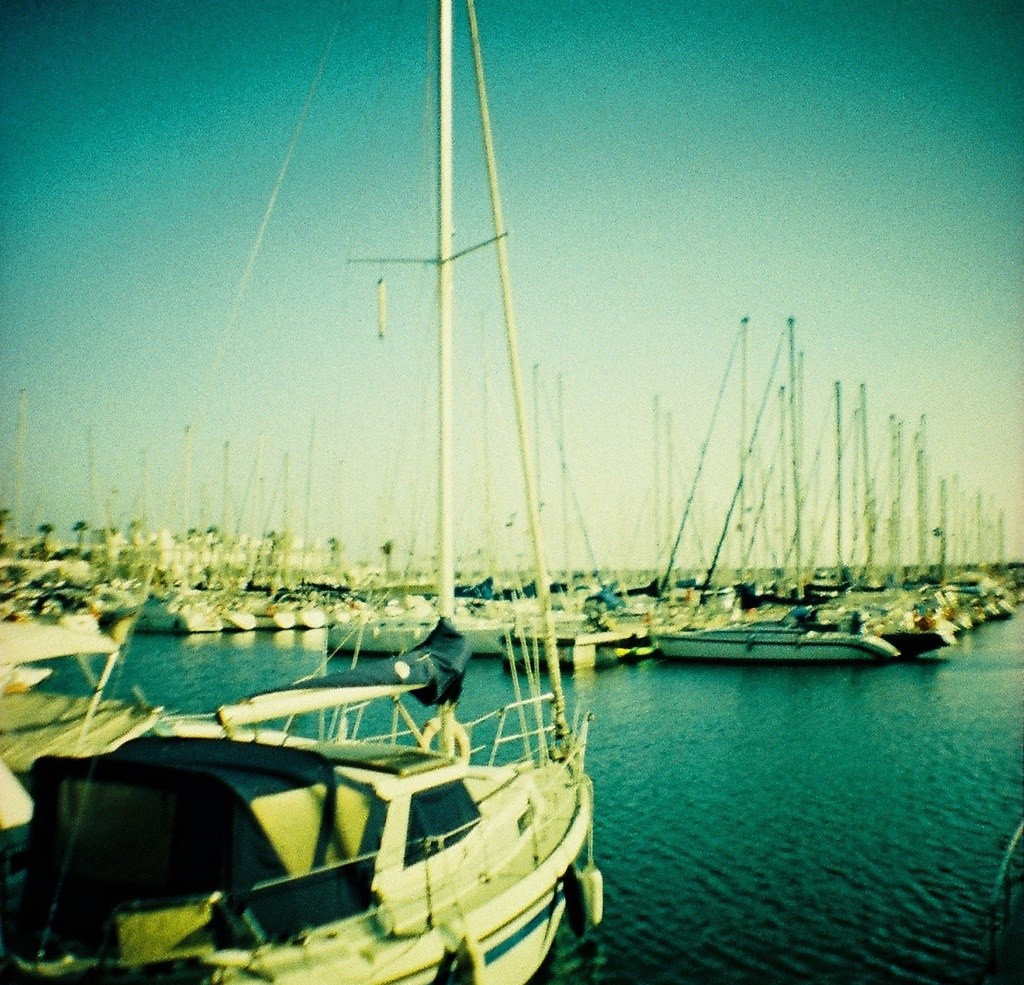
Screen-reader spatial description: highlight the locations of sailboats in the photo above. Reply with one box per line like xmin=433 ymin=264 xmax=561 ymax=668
xmin=0 ymin=2 xmax=1022 ymax=985
